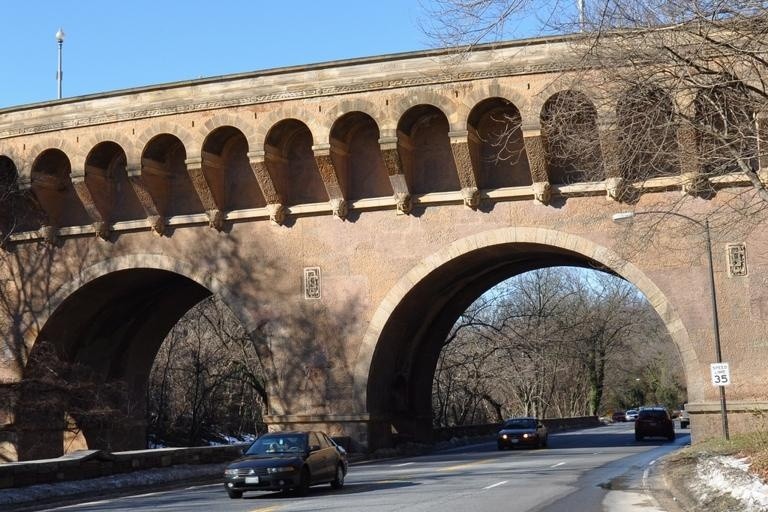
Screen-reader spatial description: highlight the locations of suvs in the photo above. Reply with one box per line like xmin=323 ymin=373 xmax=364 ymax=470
xmin=633 ymin=407 xmax=676 ymax=441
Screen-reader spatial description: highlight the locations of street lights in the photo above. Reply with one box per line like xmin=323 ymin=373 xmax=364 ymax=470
xmin=609 ymin=208 xmax=730 ymax=443
xmin=55 ymin=26 xmax=65 ymax=101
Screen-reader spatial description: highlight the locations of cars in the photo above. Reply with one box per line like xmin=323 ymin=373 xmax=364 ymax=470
xmin=496 ymin=417 xmax=549 ymax=451
xmin=612 ymin=403 xmax=691 ymax=429
xmin=224 ymin=430 xmax=349 ymax=499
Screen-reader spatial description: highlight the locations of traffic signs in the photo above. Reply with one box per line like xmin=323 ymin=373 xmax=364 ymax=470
xmin=708 ymin=362 xmax=730 ymax=387
